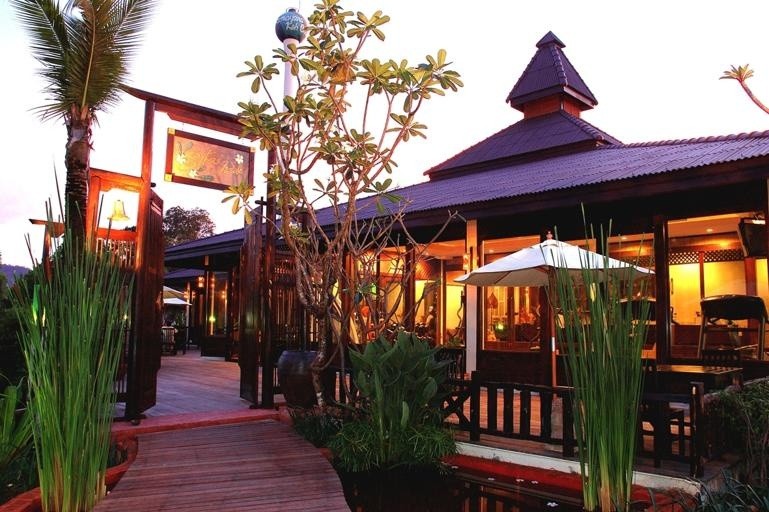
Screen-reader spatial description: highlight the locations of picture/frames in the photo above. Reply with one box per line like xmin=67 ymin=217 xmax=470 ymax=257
xmin=167 ymin=127 xmax=256 ymax=194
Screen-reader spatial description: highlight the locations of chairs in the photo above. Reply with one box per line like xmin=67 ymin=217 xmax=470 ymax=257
xmin=639 ymin=359 xmax=687 ymax=462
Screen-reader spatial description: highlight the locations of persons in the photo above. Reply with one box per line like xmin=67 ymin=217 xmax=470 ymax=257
xmin=161 ymin=321 xmax=179 ymax=354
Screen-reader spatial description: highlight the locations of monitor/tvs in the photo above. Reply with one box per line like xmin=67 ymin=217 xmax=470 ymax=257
xmin=738 ymin=216 xmax=767 ymax=258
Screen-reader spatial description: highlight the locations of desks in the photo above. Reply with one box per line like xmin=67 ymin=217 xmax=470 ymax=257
xmin=641 ymin=363 xmax=745 ymax=392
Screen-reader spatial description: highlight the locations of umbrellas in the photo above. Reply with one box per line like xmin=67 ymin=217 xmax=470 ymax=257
xmin=163 ymin=298 xmax=192 ymax=306
xmin=163 ymin=285 xmax=187 ymax=298
xmin=452 ymin=229 xmax=656 ymax=401
